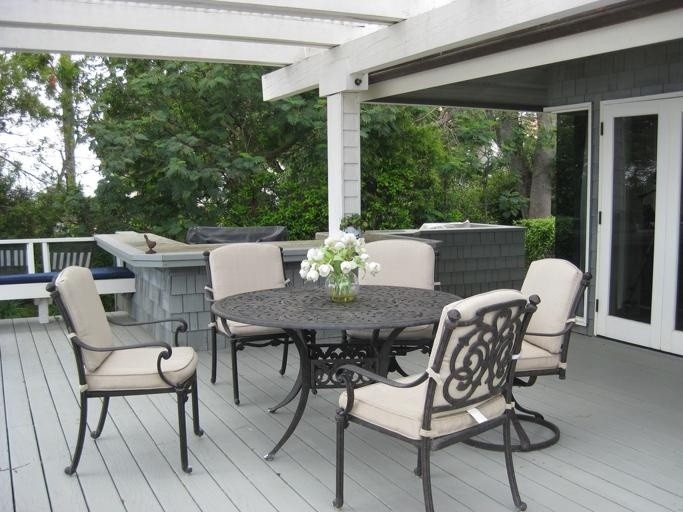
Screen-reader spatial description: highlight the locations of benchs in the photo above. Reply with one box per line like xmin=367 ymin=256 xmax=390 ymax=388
xmin=0 ymin=234 xmax=136 ymax=324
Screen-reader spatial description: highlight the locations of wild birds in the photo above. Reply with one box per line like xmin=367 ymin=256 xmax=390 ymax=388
xmin=144 ymin=235 xmax=157 ymax=253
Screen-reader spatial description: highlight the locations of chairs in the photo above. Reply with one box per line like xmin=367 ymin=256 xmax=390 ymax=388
xmin=330 ymin=287 xmax=540 ymax=512
xmin=457 ymin=256 xmax=594 ymax=453
xmin=48 ymin=264 xmax=206 ymax=477
xmin=202 ymin=242 xmax=318 ymax=405
xmin=342 ymin=235 xmax=436 ymax=378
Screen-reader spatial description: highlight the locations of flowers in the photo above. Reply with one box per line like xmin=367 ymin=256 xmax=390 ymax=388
xmin=300 ymin=232 xmax=381 ymax=303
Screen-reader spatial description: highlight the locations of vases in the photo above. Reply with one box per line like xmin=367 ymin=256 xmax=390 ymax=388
xmin=323 ymin=273 xmax=359 ymax=303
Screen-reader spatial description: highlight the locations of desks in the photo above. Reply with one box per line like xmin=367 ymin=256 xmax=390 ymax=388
xmin=203 ymin=283 xmax=465 ymax=461
